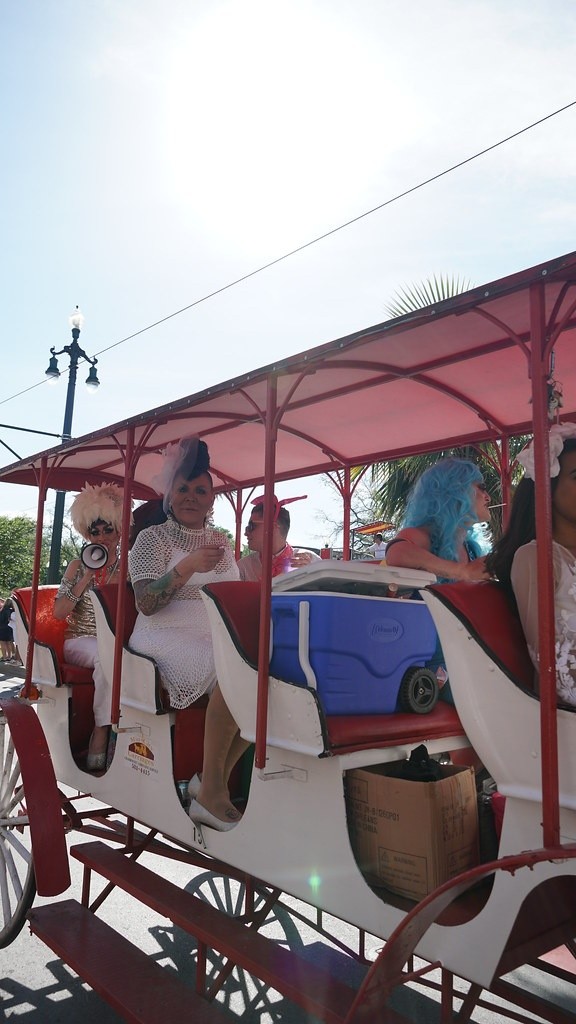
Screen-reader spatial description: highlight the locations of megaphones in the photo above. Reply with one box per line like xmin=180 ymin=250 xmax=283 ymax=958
xmin=80 ymin=543 xmax=109 ymax=569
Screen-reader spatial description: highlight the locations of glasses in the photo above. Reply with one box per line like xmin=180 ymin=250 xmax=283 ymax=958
xmin=88 ymin=525 xmax=114 ymax=536
xmin=249 ymin=520 xmax=280 ymax=532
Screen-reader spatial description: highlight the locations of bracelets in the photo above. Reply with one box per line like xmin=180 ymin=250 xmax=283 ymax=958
xmin=65 ymin=591 xmax=81 ymax=603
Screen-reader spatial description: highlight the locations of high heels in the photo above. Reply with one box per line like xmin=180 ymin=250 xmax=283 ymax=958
xmin=189 ymin=798 xmax=239 ymax=850
xmin=187 ymin=772 xmax=201 ymax=802
xmin=87 ymin=732 xmax=106 ymax=770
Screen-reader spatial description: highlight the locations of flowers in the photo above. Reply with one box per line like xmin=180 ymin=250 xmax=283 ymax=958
xmin=516 ymin=421 xmax=576 ymax=482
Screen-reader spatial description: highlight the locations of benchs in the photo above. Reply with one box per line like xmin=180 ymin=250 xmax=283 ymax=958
xmin=200 ymin=580 xmax=477 ymax=760
xmin=88 ymin=585 xmax=242 ymax=726
xmin=415 ymin=580 xmax=576 ymax=810
xmin=12 ymin=585 xmax=113 ymax=698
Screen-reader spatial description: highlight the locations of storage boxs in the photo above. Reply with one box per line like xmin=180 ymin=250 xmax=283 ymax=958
xmin=270 ymin=559 xmax=443 ymax=714
xmin=340 ymin=758 xmax=478 ymax=901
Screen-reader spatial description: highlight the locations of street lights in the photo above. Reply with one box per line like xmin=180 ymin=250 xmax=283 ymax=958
xmin=45 ymin=305 xmax=103 ymax=581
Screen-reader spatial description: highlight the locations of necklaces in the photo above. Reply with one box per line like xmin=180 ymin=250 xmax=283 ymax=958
xmin=106 ymin=559 xmax=118 ymax=584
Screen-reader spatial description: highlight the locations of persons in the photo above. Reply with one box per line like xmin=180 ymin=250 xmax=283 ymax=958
xmin=386 ymin=460 xmax=496 ymax=600
xmin=235 ymin=503 xmax=322 ymax=581
xmin=53 ymin=519 xmax=130 ymax=773
xmin=483 ymin=423 xmax=576 ymax=707
xmin=360 ymin=534 xmax=387 ymax=558
xmin=127 ymin=437 xmax=254 ymax=847
xmin=0 ymin=598 xmax=21 ymax=662
